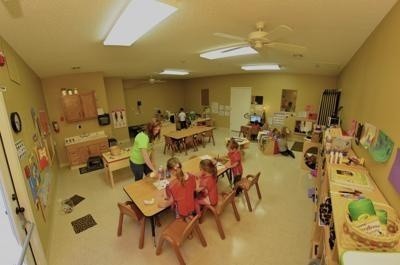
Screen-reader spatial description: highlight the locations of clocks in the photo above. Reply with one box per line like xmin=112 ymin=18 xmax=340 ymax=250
xmin=11 ymin=112 xmax=21 ymax=132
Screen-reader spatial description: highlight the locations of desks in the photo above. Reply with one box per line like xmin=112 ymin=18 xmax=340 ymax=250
xmin=122 ymin=154 xmax=231 ymax=218
xmin=101 ymin=148 xmax=131 ymax=189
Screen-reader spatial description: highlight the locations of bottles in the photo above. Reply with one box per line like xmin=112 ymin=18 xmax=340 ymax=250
xmin=330 ymin=151 xmax=342 ymax=164
xmin=158 ymin=165 xmax=165 ymax=180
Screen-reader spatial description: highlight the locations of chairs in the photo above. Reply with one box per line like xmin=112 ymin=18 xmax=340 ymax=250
xmin=116 ymin=201 xmax=162 ymax=249
xmin=155 ymin=214 xmax=208 ymax=265
xmin=233 ymin=171 xmax=263 ymax=212
xmin=162 ymin=124 xmax=216 ymax=156
xmin=199 ymin=191 xmax=241 ymax=241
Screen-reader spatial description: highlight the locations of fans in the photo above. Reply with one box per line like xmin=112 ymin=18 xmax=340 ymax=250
xmin=141 ymin=75 xmax=166 ymax=85
xmin=213 ymin=21 xmax=305 ymax=55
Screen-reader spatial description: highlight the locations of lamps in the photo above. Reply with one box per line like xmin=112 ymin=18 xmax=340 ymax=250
xmin=103 ymin=0 xmax=177 ymax=47
xmin=159 ymin=68 xmax=190 ymax=76
xmin=240 ymin=64 xmax=281 ymax=72
xmin=200 ymin=45 xmax=258 ymax=60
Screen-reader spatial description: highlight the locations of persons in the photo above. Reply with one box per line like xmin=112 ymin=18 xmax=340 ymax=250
xmin=250 ymin=119 xmax=263 ymax=142
xmin=177 ymin=107 xmax=188 ymax=129
xmin=277 ymin=133 xmax=296 ymax=159
xmin=222 ymin=137 xmax=244 ymax=196
xmin=194 ymin=158 xmax=219 ymax=223
xmin=270 ymin=127 xmax=278 ymax=137
xmin=164 ymin=156 xmax=197 ymax=219
xmin=128 ymin=118 xmax=162 ymax=182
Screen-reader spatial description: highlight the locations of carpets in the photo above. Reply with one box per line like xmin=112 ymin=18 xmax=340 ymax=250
xmin=70 ymin=213 xmax=98 ymax=235
xmin=64 ymin=194 xmax=85 ymax=208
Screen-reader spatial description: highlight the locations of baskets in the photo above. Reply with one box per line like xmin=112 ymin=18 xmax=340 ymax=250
xmin=346 ymin=201 xmax=397 ymax=251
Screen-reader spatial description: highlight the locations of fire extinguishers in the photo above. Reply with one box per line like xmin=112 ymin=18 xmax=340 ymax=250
xmin=52 ymin=121 xmax=59 ymax=132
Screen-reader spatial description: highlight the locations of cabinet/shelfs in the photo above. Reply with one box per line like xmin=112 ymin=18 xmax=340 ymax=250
xmin=59 ymin=89 xmax=98 ymax=123
xmin=309 ymin=127 xmax=400 ymax=265
xmin=64 ymin=130 xmax=109 ymax=170
